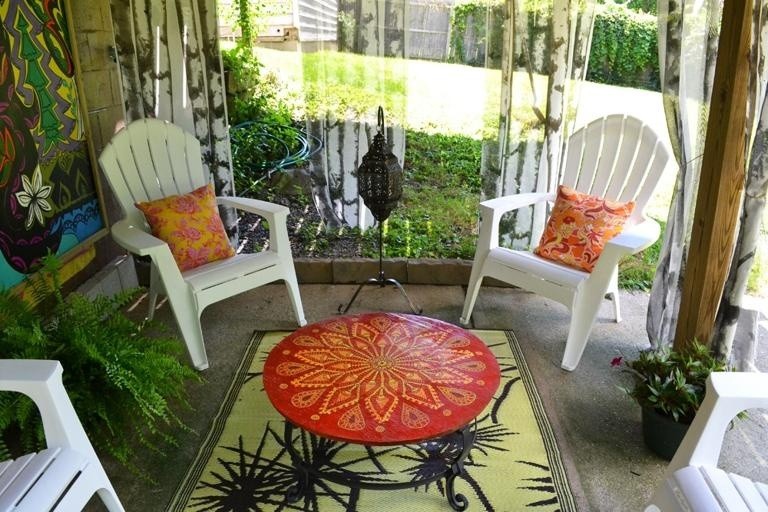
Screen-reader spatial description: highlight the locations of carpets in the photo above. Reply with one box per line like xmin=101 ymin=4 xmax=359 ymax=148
xmin=160 ymin=326 xmax=579 ymax=512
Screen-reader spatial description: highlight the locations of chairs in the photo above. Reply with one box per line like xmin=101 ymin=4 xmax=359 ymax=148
xmin=98 ymin=116 xmax=306 ymax=370
xmin=460 ymin=115 xmax=668 ymax=370
xmin=0 ymin=357 xmax=123 ymax=512
xmin=637 ymin=371 xmax=768 ymax=511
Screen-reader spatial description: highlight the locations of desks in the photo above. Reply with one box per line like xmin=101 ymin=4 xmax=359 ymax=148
xmin=264 ymin=311 xmax=502 ymax=512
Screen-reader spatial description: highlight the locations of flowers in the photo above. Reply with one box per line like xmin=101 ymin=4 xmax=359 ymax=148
xmin=610 ymin=340 xmax=749 ymax=424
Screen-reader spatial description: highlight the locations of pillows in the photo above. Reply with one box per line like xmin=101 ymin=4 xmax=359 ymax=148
xmin=534 ymin=187 xmax=634 ymax=273
xmin=134 ymin=185 xmax=236 ymax=267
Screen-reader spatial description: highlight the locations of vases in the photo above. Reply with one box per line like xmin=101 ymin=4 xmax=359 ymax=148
xmin=642 ymin=406 xmax=692 ymax=460
